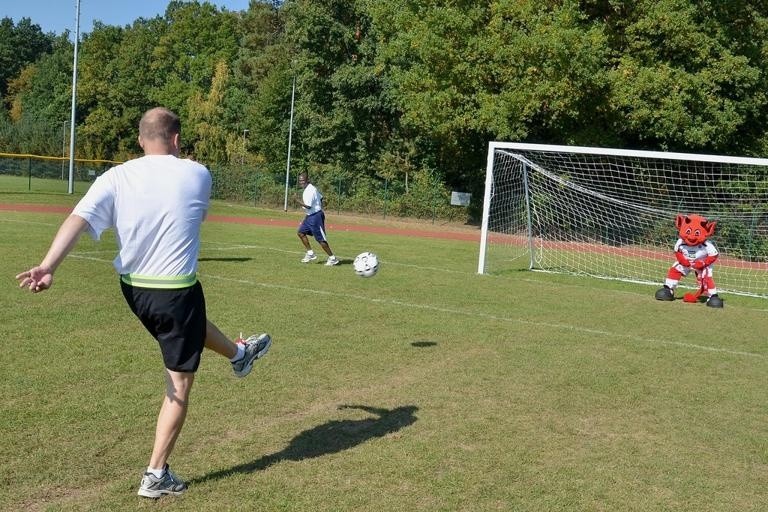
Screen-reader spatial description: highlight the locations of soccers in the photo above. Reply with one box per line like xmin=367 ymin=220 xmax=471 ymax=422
xmin=353 ymin=251 xmax=379 ymax=278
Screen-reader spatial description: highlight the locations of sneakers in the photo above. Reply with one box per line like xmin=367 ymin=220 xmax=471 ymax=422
xmin=137 ymin=462 xmax=187 ymax=498
xmin=230 ymin=333 xmax=271 ymax=378
xmin=301 ymin=252 xmax=317 ymax=263
xmin=324 ymin=256 xmax=339 ymax=266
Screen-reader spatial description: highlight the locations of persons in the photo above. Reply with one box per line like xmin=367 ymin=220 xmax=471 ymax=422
xmin=16 ymin=104 xmax=273 ymax=502
xmin=291 ymin=172 xmax=341 ymax=267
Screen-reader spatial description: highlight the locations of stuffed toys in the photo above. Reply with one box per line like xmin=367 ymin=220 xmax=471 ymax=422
xmin=653 ymin=211 xmax=724 ymax=309
